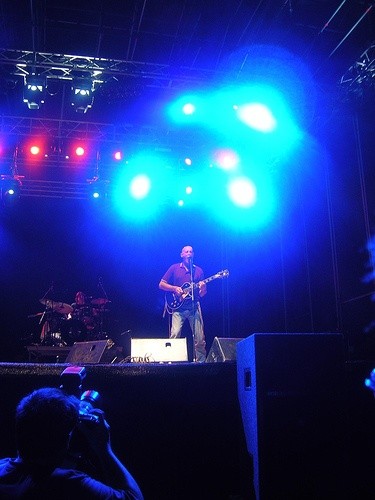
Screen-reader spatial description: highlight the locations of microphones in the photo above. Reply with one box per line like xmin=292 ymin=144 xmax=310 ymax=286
xmin=187 ymin=256 xmax=192 ymax=260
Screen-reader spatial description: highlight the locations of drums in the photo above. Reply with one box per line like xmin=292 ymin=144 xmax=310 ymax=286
xmin=49 ymin=308 xmax=95 ymax=343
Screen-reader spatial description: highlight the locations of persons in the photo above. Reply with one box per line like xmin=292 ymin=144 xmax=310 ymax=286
xmin=0 ymin=387 xmax=144 ymax=500
xmin=158 ymin=245 xmax=207 ymax=363
xmin=71 ymin=291 xmax=84 ymax=310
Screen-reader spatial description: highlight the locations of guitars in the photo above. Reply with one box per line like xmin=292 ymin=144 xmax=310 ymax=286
xmin=164 ymin=269 xmax=230 ymax=311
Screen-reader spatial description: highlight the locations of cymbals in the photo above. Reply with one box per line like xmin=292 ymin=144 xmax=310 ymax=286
xmin=91 ymin=297 xmax=108 ymax=305
xmin=40 ymin=299 xmax=73 ymax=314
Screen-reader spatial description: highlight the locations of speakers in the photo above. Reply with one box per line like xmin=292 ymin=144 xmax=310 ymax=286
xmin=132 ymin=337 xmax=188 ymax=364
xmin=236 ymin=328 xmax=346 ymax=500
xmin=205 ymin=336 xmax=245 ymax=363
xmin=64 ymin=340 xmax=129 ymax=364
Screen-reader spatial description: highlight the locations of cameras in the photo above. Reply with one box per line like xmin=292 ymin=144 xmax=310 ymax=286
xmin=72 ymin=390 xmax=103 ymax=447
